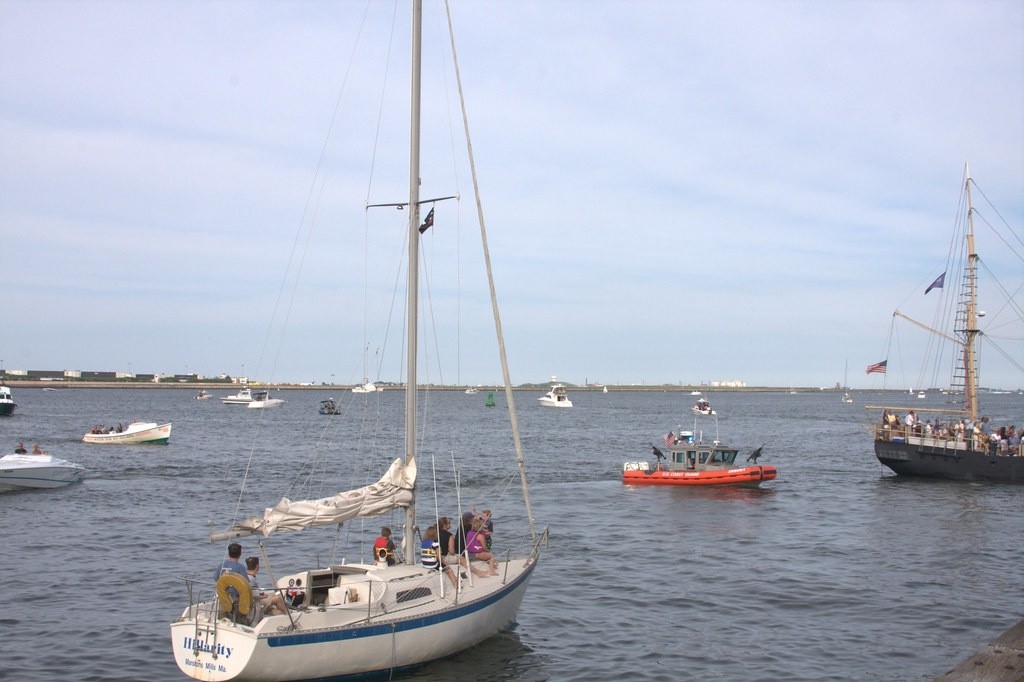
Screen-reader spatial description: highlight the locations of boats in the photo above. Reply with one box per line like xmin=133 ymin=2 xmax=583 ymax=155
xmin=42 ymin=387 xmax=59 ymax=395
xmin=0 ymin=377 xmax=18 ymax=417
xmin=318 ymin=398 xmax=341 ymax=415
xmin=0 ymin=452 xmax=87 ymax=495
xmin=247 ymin=391 xmax=286 ymax=409
xmin=596 ymin=383 xmax=1024 ymax=411
xmin=486 ymin=391 xmax=496 ymax=406
xmin=537 ymin=384 xmax=573 ymax=408
xmin=464 ymin=388 xmax=480 ymax=395
xmin=83 ymin=417 xmax=172 ymax=444
xmin=622 ymin=391 xmax=778 ymax=487
xmin=194 ymin=391 xmax=213 ymax=400
xmin=219 ymin=389 xmax=254 ymax=404
xmin=351 ymin=381 xmax=390 ymax=396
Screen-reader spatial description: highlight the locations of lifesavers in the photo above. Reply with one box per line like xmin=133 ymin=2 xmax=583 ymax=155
xmin=216 ymin=572 xmax=252 ymax=615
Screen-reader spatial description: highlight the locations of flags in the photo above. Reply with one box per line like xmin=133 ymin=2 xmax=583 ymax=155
xmin=418 ymin=207 xmax=434 ymax=235
xmin=924 ymin=271 xmax=946 ymax=294
xmin=664 ymin=431 xmax=675 ymax=447
xmin=866 ymin=360 xmax=887 ymax=374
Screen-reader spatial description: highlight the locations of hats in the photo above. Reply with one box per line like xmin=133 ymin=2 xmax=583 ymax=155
xmin=462 ymin=512 xmax=473 ymax=518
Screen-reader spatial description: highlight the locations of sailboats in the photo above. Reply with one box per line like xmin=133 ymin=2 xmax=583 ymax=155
xmin=864 ymin=161 xmax=1024 ymax=483
xmin=171 ymin=1 xmax=552 ymax=682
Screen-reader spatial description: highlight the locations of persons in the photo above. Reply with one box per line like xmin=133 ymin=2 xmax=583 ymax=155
xmin=15 ymin=443 xmax=48 ymax=455
xmin=883 ymin=409 xmax=1024 ymax=455
xmin=92 ymin=423 xmax=122 ymax=434
xmin=372 ymin=526 xmax=397 ymax=566
xmin=214 ymin=544 xmax=293 ymax=626
xmin=687 ymin=455 xmax=695 ymax=469
xmin=421 ymin=509 xmax=499 ymax=590
